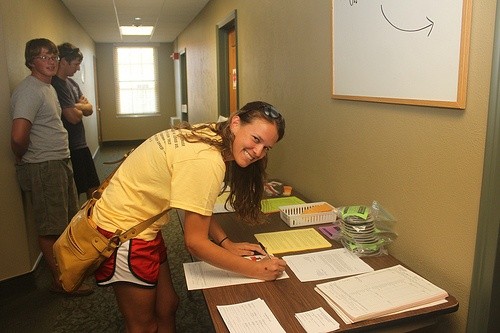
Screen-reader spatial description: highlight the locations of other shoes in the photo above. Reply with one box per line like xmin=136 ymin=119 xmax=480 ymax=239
xmin=59 ymin=283 xmax=94 ymax=296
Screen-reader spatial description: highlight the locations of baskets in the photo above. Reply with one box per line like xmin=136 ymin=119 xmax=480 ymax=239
xmin=279 ymin=200 xmax=338 ymax=227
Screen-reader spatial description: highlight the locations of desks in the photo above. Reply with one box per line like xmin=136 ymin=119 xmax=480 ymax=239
xmin=176 ymin=179 xmax=459 ymax=333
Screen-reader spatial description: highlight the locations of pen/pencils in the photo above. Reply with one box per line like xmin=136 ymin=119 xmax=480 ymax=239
xmin=257 ymin=241 xmax=272 ymax=261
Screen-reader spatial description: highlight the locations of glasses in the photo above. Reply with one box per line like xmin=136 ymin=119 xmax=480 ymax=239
xmin=60 ymin=48 xmax=80 ymax=60
xmin=239 ymin=104 xmax=286 ymax=129
xmin=31 ymin=55 xmax=60 ymax=62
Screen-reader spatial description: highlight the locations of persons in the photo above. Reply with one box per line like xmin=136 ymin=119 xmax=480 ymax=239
xmin=96 ymin=100 xmax=285 ymax=333
xmin=10 ymin=38 xmax=101 ymax=296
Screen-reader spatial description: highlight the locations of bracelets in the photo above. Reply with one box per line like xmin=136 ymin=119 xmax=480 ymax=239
xmin=220 ymin=236 xmax=229 ymax=246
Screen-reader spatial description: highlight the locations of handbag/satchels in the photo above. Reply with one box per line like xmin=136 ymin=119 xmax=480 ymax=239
xmin=52 ymin=197 xmax=120 ymax=295
